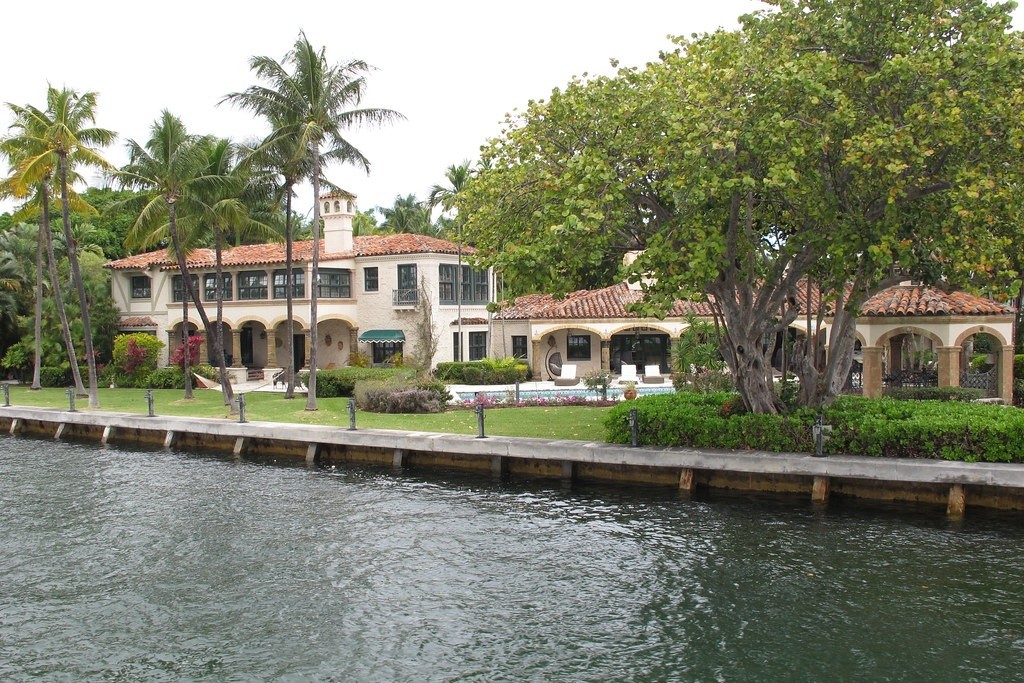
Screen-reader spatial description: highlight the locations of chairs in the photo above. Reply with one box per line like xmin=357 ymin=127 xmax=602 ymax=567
xmin=325 ymin=363 xmax=335 ymax=370
xmin=555 ymin=364 xmax=581 ymax=386
xmin=687 ymin=364 xmax=710 ymax=381
xmin=642 ymin=365 xmax=664 ymax=383
xmin=588 ymin=374 xmax=612 ymax=384
xmin=617 ymin=364 xmax=641 ymax=385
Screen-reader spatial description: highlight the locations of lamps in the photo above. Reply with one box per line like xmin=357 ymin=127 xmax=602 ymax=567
xmin=346 ymin=401 xmax=353 ymax=413
xmin=144 ymin=393 xmax=151 ymax=402
xmin=474 ymin=405 xmax=482 ymax=417
xmin=814 ymin=418 xmax=822 ymax=434
xmin=64 ymin=388 xmax=72 ymax=399
xmin=235 ymin=396 xmax=242 ymax=408
xmin=0 ymin=386 xmax=7 ymax=394
xmin=627 ymin=411 xmax=637 ymax=427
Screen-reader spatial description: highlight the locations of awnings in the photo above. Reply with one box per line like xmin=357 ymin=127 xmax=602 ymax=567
xmin=358 ymin=329 xmax=406 ymax=344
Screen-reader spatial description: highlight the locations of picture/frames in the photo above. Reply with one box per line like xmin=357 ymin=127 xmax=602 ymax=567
xmin=624 ymin=328 xmax=630 ymax=331
xmin=633 ymin=327 xmax=639 ymax=331
xmin=641 ymin=327 xmax=647 ymax=331
xmin=650 ymin=328 xmax=656 ymax=331
